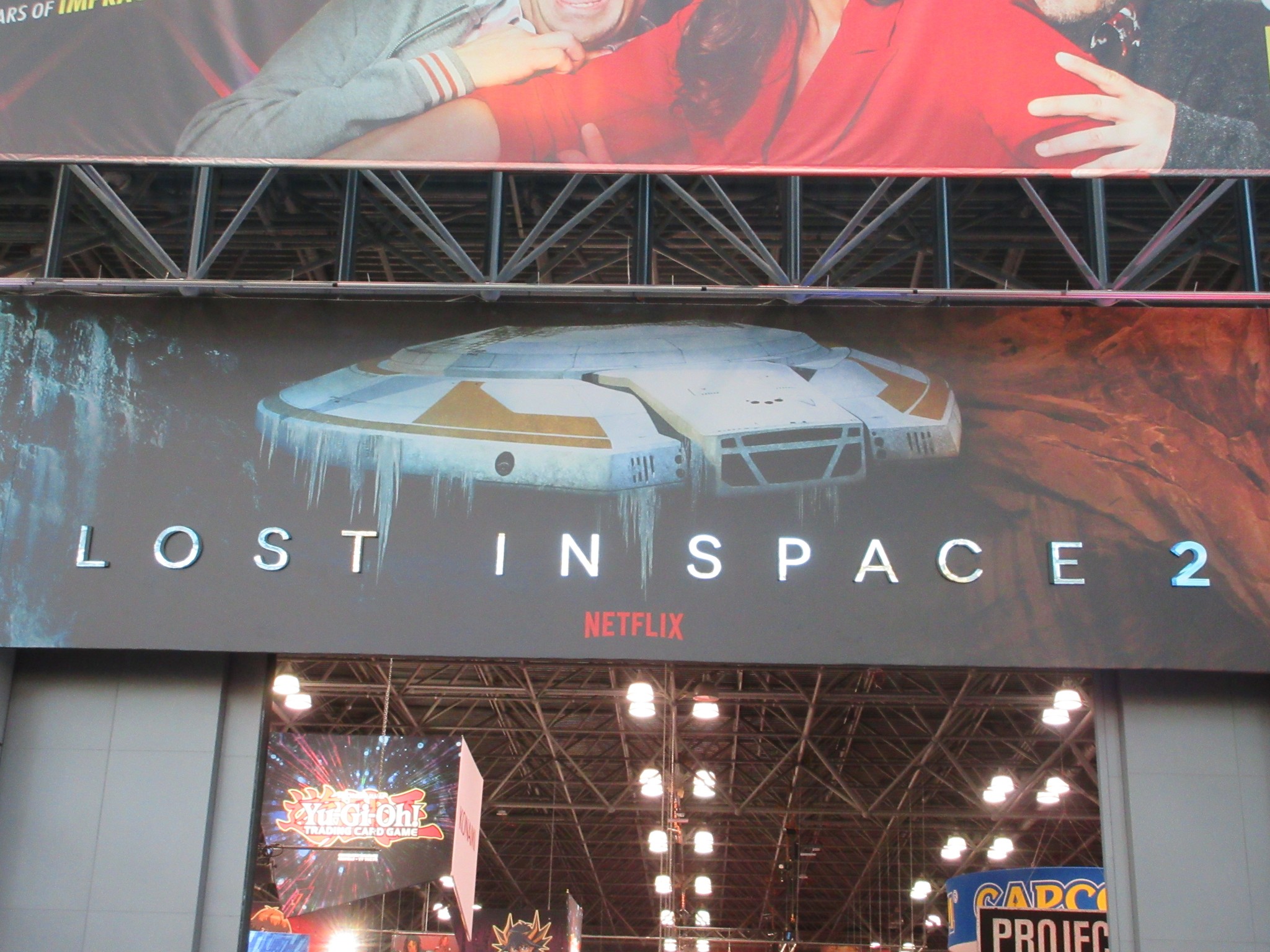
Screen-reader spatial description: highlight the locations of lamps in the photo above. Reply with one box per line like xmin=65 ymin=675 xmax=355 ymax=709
xmin=1052 ymin=675 xmax=1083 ymax=710
xmin=691 ymin=672 xmax=720 ymax=702
xmin=627 ymin=667 xmax=654 ymax=702
xmin=272 ymin=659 xmax=301 ymax=695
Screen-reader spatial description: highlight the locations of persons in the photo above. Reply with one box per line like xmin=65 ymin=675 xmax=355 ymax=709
xmin=170 ymin=1 xmax=1270 ymax=175
xmin=403 ymin=935 xmax=426 ymax=952
